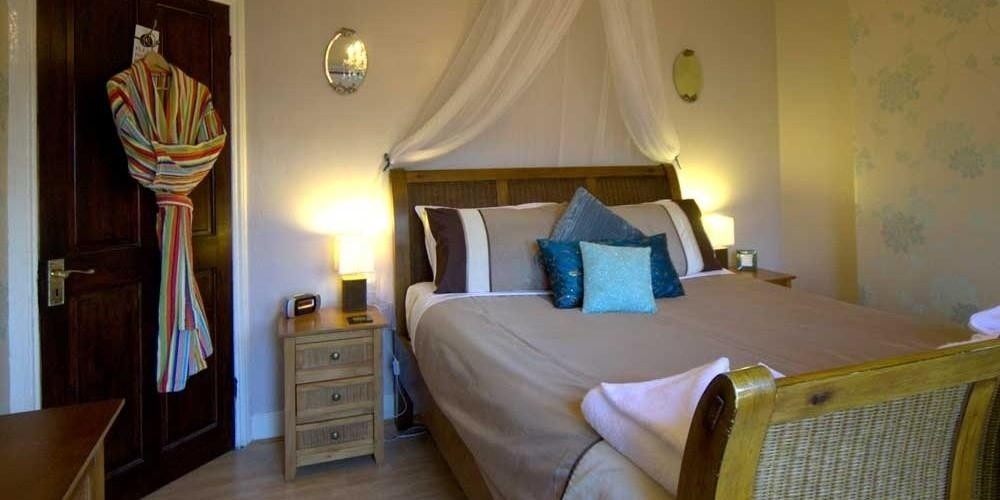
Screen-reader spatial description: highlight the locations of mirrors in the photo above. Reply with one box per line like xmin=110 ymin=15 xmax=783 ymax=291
xmin=325 ymin=27 xmax=369 ymax=95
xmin=673 ymin=48 xmax=704 ymax=103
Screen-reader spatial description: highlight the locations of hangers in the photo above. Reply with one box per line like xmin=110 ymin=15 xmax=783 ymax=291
xmin=138 ymin=31 xmax=178 ymax=97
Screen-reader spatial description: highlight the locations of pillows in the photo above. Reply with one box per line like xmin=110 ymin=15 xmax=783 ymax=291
xmin=532 ymin=188 xmax=649 ymax=280
xmin=415 ymin=199 xmax=721 ymax=294
xmin=579 ymin=242 xmax=657 ymax=314
xmin=536 ymin=233 xmax=685 ymax=309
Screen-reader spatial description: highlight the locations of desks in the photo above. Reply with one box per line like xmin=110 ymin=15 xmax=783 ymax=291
xmin=0 ymin=394 xmax=122 ymax=499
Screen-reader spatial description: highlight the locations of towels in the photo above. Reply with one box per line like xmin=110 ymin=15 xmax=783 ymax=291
xmin=581 ymin=352 xmax=788 ymax=500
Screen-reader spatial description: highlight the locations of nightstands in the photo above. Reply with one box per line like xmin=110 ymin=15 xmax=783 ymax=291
xmin=275 ymin=306 xmax=391 ymax=482
xmin=726 ymin=265 xmax=797 ymax=290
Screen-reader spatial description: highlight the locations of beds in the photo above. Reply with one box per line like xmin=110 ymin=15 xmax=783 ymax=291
xmin=388 ymin=159 xmax=1000 ymax=500
